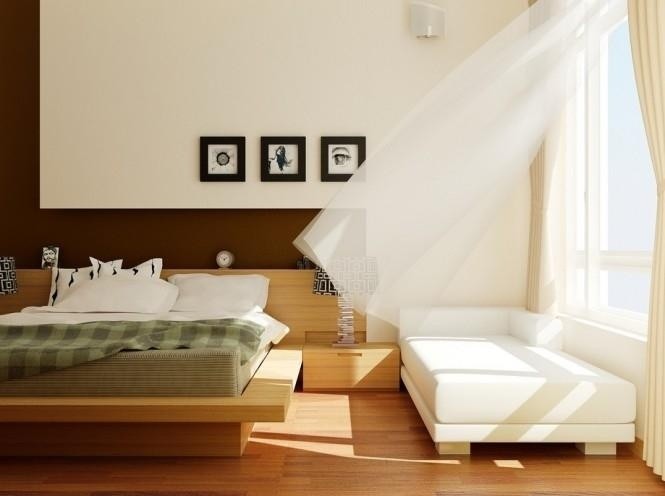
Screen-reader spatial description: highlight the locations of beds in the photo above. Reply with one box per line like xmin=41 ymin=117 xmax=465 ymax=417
xmin=0 ymin=268 xmax=366 ymax=457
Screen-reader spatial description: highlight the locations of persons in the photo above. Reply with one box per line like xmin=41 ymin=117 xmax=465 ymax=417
xmin=270 ymin=146 xmax=292 ymax=174
xmin=42 ymin=247 xmax=57 ymax=268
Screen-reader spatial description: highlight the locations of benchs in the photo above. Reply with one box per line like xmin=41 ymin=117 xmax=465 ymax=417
xmin=399 ymin=309 xmax=636 ymax=456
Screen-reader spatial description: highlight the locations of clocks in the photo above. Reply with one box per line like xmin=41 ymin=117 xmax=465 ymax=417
xmin=216 ymin=250 xmax=235 ymax=268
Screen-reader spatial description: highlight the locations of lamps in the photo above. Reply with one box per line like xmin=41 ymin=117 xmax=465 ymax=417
xmin=410 ymin=3 xmax=445 ymax=38
xmin=312 ymin=261 xmax=379 ymax=346
xmin=0 ymin=256 xmax=18 ymax=295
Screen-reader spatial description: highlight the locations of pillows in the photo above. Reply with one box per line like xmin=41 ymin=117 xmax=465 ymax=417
xmin=47 ymin=256 xmax=269 ymax=315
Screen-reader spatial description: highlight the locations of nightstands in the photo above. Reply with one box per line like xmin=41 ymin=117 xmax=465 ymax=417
xmin=302 ymin=331 xmax=400 ymax=391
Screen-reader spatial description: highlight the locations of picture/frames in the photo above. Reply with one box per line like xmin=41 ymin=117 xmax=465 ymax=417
xmin=200 ymin=136 xmax=245 ymax=182
xmin=261 ymin=137 xmax=306 ymax=182
xmin=321 ymin=136 xmax=366 ymax=182
